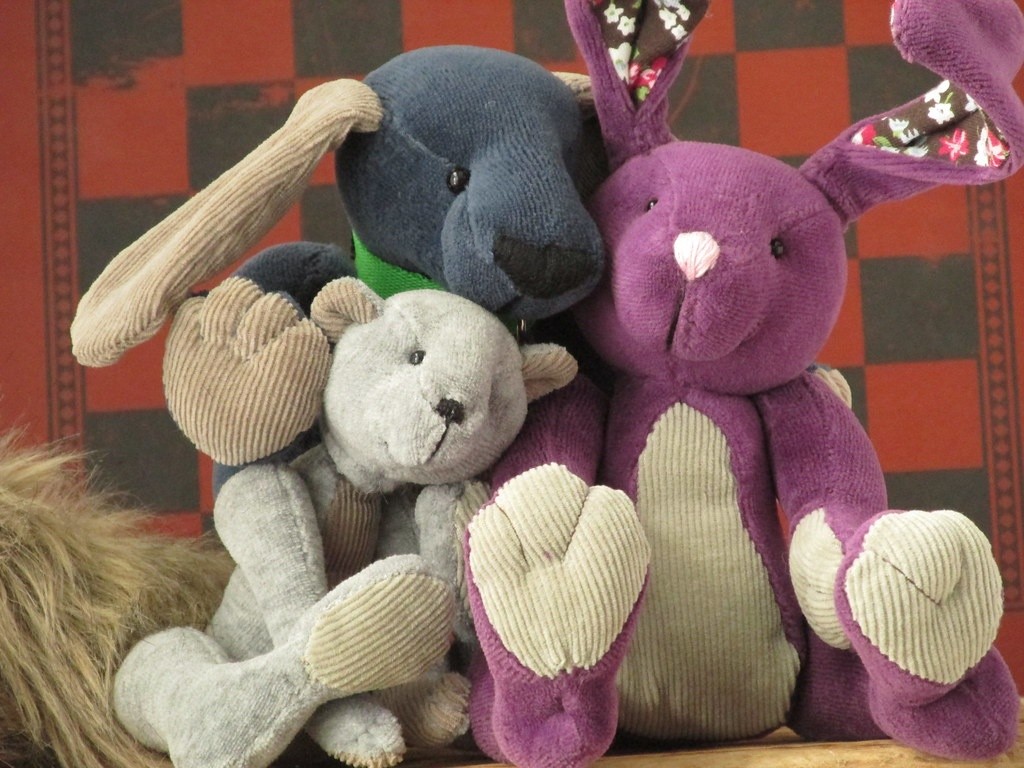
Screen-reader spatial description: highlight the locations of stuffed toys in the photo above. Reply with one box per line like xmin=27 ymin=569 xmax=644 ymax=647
xmin=108 ymin=276 xmax=582 ymax=768
xmin=67 ymin=44 xmax=610 ymax=507
xmin=458 ymin=0 xmax=1022 ymax=768
